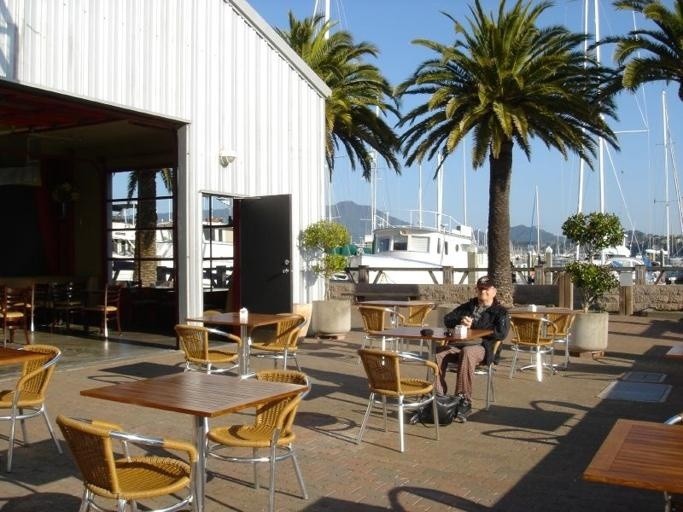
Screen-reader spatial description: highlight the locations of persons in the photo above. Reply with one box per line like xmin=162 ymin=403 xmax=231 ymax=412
xmin=427 ymin=276 xmax=511 ymax=417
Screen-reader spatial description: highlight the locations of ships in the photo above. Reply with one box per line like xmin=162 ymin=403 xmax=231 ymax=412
xmin=328 ymin=209 xmax=489 ymax=284
xmin=110 ymin=201 xmax=232 ymax=289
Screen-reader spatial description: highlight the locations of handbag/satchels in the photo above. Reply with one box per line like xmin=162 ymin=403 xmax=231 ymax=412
xmin=418 ymin=393 xmax=460 ymax=424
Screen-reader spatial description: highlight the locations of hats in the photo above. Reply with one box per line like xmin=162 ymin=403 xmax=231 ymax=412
xmin=476 ymin=275 xmax=496 ymax=289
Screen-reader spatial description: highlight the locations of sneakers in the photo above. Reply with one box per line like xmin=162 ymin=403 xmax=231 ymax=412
xmin=453 ymin=398 xmax=472 ymax=422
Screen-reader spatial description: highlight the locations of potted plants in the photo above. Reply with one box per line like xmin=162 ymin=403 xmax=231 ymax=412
xmin=298 ymin=219 xmax=352 ymax=334
xmin=563 ymin=211 xmax=624 ymax=353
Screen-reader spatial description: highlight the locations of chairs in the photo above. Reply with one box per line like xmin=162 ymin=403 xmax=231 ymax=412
xmin=401 ymin=302 xmax=435 ymax=351
xmin=357 ymin=349 xmax=441 ymax=452
xmin=0 ymin=345 xmax=63 ymax=475
xmin=51 ymin=287 xmax=83 ymax=329
xmin=440 ymin=339 xmax=502 ymax=411
xmin=250 ymin=312 xmax=309 ymax=372
xmin=26 ymin=283 xmax=50 ymax=328
xmin=57 ymin=415 xmax=197 ymax=512
xmin=542 ymin=308 xmax=571 ymax=369
xmin=200 ymin=371 xmax=310 ymax=512
xmin=357 ymin=306 xmax=404 ymax=362
xmin=508 ymin=315 xmax=553 ymax=380
xmin=81 ymin=282 xmax=122 ymax=335
xmin=665 ymin=346 xmax=683 ymax=361
xmin=174 ymin=325 xmax=241 ymax=375
xmin=0 ymin=280 xmax=36 ymax=346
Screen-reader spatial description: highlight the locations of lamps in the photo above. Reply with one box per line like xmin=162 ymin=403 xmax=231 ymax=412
xmin=221 ymin=151 xmax=238 ymax=167
